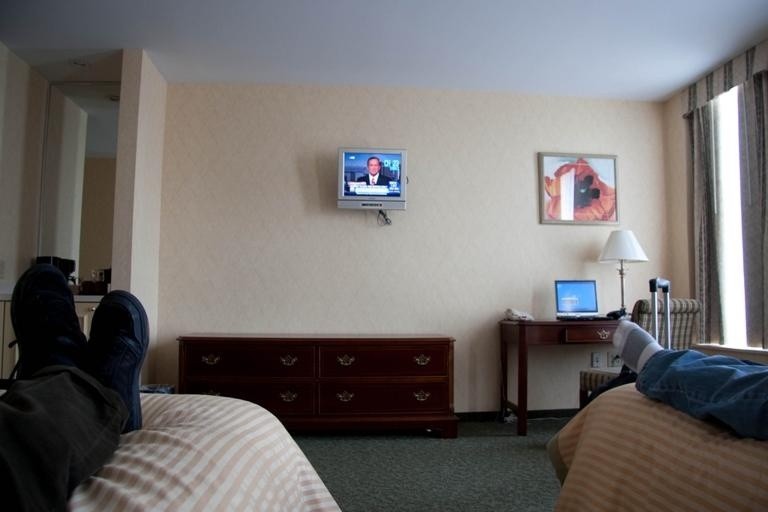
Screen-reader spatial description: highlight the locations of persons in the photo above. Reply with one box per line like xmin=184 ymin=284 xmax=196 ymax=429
xmin=610 ymin=316 xmax=768 ymax=443
xmin=356 ymin=157 xmax=391 ymax=186
xmin=0 ymin=264 xmax=148 ymax=512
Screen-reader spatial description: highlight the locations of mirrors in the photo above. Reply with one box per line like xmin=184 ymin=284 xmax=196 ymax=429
xmin=38 ymin=79 xmax=121 ymax=291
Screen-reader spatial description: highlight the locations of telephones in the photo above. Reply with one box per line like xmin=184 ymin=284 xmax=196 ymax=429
xmin=504 ymin=308 xmax=534 ymax=321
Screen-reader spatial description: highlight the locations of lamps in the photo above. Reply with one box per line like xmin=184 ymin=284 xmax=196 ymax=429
xmin=598 ymin=229 xmax=651 ymax=312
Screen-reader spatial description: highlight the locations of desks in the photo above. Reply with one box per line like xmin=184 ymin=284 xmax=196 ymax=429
xmin=497 ymin=318 xmax=630 ymax=438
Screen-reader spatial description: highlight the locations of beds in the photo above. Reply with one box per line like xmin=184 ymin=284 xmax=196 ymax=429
xmin=0 ymin=387 xmax=345 ymax=512
xmin=544 ymin=378 xmax=768 ymax=511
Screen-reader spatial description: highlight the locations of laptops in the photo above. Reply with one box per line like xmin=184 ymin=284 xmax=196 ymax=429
xmin=554 ymin=279 xmax=610 ymax=321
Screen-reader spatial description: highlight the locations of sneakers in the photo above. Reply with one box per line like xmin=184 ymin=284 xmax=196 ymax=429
xmin=89 ymin=290 xmax=150 ymax=430
xmin=11 ymin=264 xmax=88 ymax=384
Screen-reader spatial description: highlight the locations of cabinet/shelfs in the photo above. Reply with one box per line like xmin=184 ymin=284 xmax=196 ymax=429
xmin=1 ymin=293 xmax=104 ymax=380
xmin=176 ymin=334 xmax=317 ymax=429
xmin=318 ymin=335 xmax=456 ymax=437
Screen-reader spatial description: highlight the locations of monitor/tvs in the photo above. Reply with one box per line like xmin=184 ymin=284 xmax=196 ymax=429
xmin=337 ymin=148 xmax=408 ymax=211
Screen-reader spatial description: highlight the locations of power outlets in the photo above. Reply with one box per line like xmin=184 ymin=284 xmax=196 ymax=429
xmin=591 ymin=351 xmax=603 ymax=368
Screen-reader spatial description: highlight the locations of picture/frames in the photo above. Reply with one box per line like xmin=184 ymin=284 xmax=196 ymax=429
xmin=535 ymin=151 xmax=622 ymax=226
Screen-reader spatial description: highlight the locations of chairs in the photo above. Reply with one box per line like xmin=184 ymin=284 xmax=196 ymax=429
xmin=578 ymin=298 xmax=702 ymax=416
